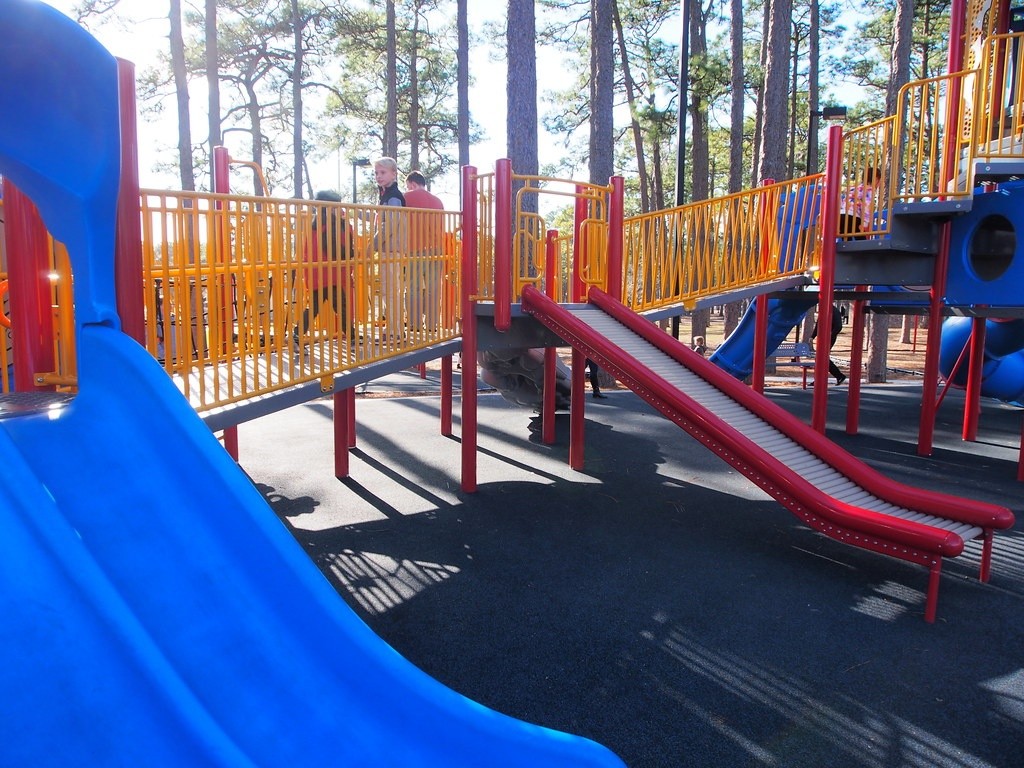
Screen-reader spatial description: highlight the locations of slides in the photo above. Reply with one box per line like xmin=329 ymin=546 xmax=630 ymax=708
xmin=706 ymin=285 xmax=817 ymax=380
xmin=940 ymin=307 xmax=1023 ymax=405
xmin=1 ymin=324 xmax=627 ymax=768
xmin=526 ymin=279 xmax=1014 ymax=560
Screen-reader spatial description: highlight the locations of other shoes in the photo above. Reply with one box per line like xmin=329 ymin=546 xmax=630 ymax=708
xmin=405 ymin=329 xmax=436 ymax=332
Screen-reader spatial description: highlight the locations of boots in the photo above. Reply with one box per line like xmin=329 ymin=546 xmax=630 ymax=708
xmin=593 ymin=387 xmax=607 ymax=398
xmin=831 ymin=367 xmax=846 ymax=386
xmin=807 ymin=381 xmax=814 ymax=386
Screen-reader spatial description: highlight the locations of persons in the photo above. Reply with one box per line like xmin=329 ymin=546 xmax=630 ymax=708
xmin=402 ymin=170 xmax=445 ymax=332
xmin=833 ymin=301 xmax=849 ymax=325
xmin=839 ymin=166 xmax=882 ymax=242
xmin=693 ymin=335 xmax=708 ymax=357
xmin=283 ymin=190 xmax=368 ymax=357
xmin=585 ymin=357 xmax=607 ymax=398
xmin=457 ymin=353 xmax=462 ymax=369
xmin=808 ymin=301 xmax=847 ymax=386
xmin=349 ymin=156 xmax=409 ymax=344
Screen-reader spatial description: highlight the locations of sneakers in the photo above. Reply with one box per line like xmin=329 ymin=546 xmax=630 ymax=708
xmin=352 ymin=335 xmax=370 ymax=344
xmin=284 ymin=333 xmax=308 ymax=355
xmin=375 ymin=332 xmax=406 ymax=345
